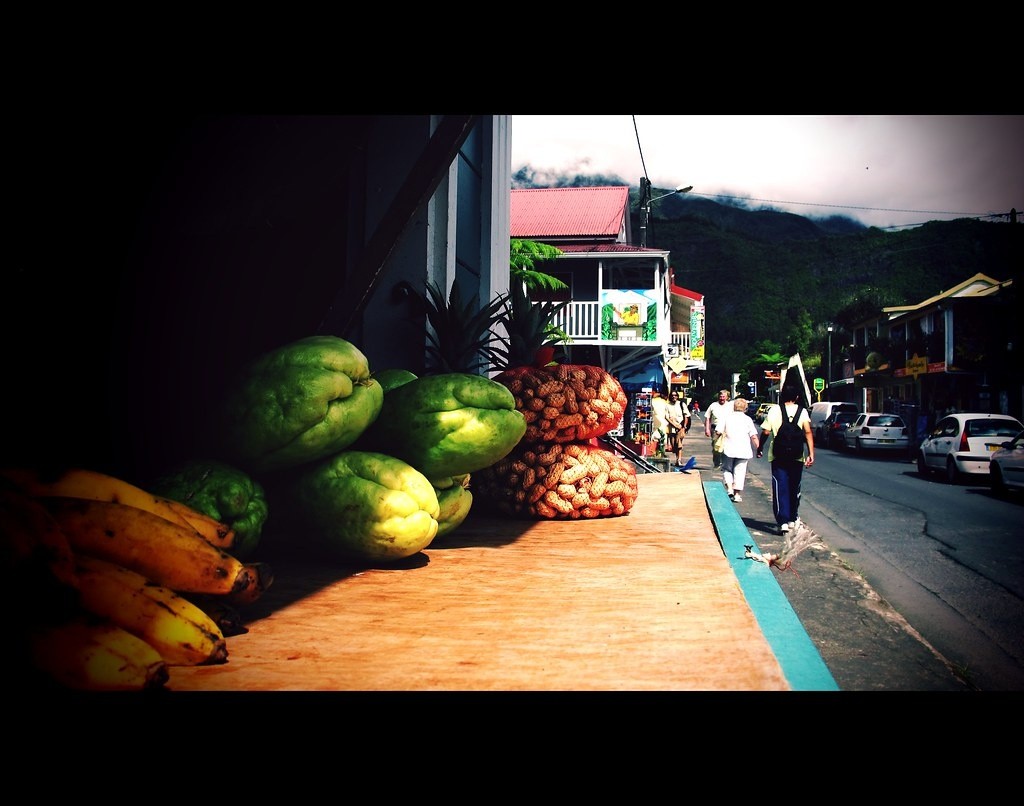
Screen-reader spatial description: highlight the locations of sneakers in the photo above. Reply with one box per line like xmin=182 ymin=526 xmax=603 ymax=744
xmin=776 ymin=523 xmax=789 ymax=536
xmin=788 ymin=521 xmax=795 ymax=529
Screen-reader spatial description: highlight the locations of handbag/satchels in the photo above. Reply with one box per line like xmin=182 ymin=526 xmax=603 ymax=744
xmin=679 ymin=400 xmax=692 ymax=433
xmin=713 ymin=432 xmax=727 ymax=454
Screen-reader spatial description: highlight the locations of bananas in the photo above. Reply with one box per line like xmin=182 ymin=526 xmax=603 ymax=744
xmin=0 ymin=469 xmax=272 ymax=691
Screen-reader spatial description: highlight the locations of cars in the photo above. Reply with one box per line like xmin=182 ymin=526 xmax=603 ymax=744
xmin=917 ymin=412 xmax=1024 ymax=485
xmin=989 ymin=429 xmax=1024 ymax=496
xmin=843 ymin=411 xmax=911 ymax=458
xmin=744 ymin=401 xmax=777 ymax=424
xmin=818 ymin=410 xmax=859 ymax=449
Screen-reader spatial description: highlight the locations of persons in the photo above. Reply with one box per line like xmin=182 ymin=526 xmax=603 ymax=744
xmin=694 ymin=401 xmax=699 ymax=415
xmin=757 ymin=379 xmax=814 ymax=532
xmin=704 ymin=389 xmax=730 ymax=470
xmin=664 ymin=390 xmax=692 ymax=466
xmin=716 ymin=399 xmax=759 ymax=503
xmin=651 ymin=393 xmax=669 ymax=446
xmin=613 ymin=303 xmax=639 ymax=325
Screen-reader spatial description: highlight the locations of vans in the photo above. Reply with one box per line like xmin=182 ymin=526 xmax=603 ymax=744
xmin=806 ymin=400 xmax=859 ymax=438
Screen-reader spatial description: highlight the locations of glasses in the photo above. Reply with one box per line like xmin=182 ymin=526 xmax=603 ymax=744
xmin=671 ymin=395 xmax=678 ymax=397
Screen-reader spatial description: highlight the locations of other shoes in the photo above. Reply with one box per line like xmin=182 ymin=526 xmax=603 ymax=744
xmin=733 ymin=495 xmax=743 ymax=502
xmin=675 ymin=459 xmax=684 ymax=467
xmin=727 ymin=489 xmax=734 ymax=498
xmin=713 ymin=464 xmax=721 ymax=471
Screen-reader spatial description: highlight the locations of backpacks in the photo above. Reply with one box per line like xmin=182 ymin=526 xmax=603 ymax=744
xmin=772 ymin=403 xmax=805 ymax=461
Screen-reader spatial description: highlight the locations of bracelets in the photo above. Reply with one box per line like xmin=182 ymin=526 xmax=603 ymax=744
xmin=683 ymin=426 xmax=687 ymax=429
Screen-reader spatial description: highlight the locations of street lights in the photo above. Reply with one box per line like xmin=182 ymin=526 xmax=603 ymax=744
xmin=825 ymin=325 xmax=835 ymax=403
xmin=638 ymin=185 xmax=694 ymax=247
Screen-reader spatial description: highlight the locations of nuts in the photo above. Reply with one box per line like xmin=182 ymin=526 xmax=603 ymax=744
xmin=486 ymin=366 xmax=637 ymax=520
